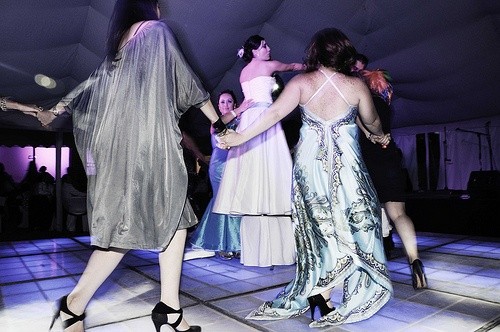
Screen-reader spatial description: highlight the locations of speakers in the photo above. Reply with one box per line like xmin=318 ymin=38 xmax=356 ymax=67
xmin=467 ymin=170 xmax=500 ymax=198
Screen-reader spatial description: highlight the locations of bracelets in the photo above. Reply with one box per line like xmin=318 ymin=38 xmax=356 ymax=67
xmin=230 ymin=110 xmax=237 ymax=118
xmin=52 ymin=104 xmax=61 ymax=118
xmin=367 ymin=133 xmax=373 ymax=140
xmin=1 ymin=96 xmax=14 ymax=112
xmin=212 ymin=116 xmax=226 ymax=137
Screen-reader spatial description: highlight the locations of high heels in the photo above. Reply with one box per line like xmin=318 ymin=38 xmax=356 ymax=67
xmin=49 ymin=295 xmax=85 ymax=332
xmin=383 ymin=232 xmax=395 ymax=261
xmin=307 ymin=293 xmax=336 ymax=321
xmin=151 ymin=301 xmax=201 ymax=332
xmin=408 ymin=259 xmax=428 ymax=289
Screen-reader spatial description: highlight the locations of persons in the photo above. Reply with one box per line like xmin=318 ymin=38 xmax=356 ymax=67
xmin=233 ymin=35 xmax=307 ymax=269
xmin=0 ymin=161 xmax=56 ymax=242
xmin=208 ymin=89 xmax=241 ymax=260
xmin=62 ymin=163 xmax=88 ymax=233
xmin=215 ymin=28 xmax=391 ymax=328
xmin=351 ymin=53 xmax=427 ymax=290
xmin=36 ymin=0 xmax=227 ymax=332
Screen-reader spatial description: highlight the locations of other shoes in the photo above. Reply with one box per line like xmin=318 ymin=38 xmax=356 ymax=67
xmin=215 ymin=251 xmax=241 ymax=261
